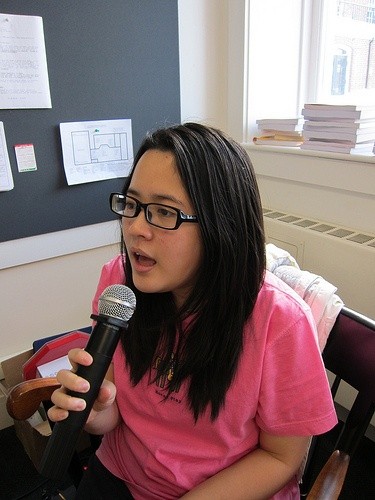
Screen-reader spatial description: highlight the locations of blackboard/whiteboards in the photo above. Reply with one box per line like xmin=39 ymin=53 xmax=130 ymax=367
xmin=0 ymin=0 xmax=183 ymax=244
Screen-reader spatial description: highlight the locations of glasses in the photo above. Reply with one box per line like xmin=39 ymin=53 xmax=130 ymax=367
xmin=108 ymin=191 xmax=198 ymax=231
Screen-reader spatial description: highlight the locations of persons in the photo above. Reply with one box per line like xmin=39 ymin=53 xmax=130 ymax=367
xmin=48 ymin=123 xmax=340 ymax=500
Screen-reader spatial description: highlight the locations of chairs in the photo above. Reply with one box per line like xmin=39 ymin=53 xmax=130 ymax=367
xmin=4 ymin=306 xmax=375 ymax=500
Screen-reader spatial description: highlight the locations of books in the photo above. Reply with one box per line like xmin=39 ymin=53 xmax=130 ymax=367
xmin=253 ymin=95 xmax=375 ymax=156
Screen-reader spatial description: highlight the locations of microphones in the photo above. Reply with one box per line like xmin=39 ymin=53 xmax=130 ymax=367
xmin=37 ymin=283 xmax=136 ymax=482
